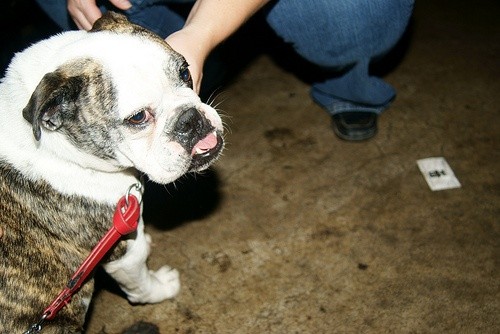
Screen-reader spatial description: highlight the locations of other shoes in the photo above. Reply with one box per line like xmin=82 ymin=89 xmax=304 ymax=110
xmin=332 ymin=111 xmax=377 ymax=142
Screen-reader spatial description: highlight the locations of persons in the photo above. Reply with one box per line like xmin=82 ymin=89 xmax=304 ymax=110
xmin=36 ymin=0 xmax=415 ymax=141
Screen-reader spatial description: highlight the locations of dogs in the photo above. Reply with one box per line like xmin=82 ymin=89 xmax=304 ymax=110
xmin=0 ymin=11 xmax=233 ymax=334
